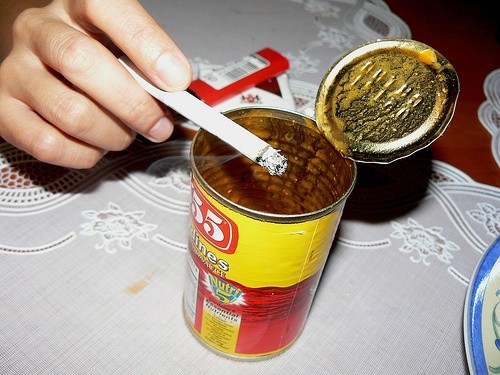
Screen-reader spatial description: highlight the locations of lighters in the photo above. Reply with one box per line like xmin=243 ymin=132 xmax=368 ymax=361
xmin=186 ymin=45 xmax=291 ymax=108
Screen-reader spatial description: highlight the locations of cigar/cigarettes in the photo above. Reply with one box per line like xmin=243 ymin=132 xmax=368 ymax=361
xmin=114 ymin=53 xmax=293 ymax=172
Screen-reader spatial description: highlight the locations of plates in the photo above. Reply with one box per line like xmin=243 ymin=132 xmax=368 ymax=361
xmin=462 ymin=235 xmax=500 ymax=375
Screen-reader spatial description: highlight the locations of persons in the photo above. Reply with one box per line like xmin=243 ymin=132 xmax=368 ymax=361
xmin=0 ymin=0 xmax=195 ymax=170
